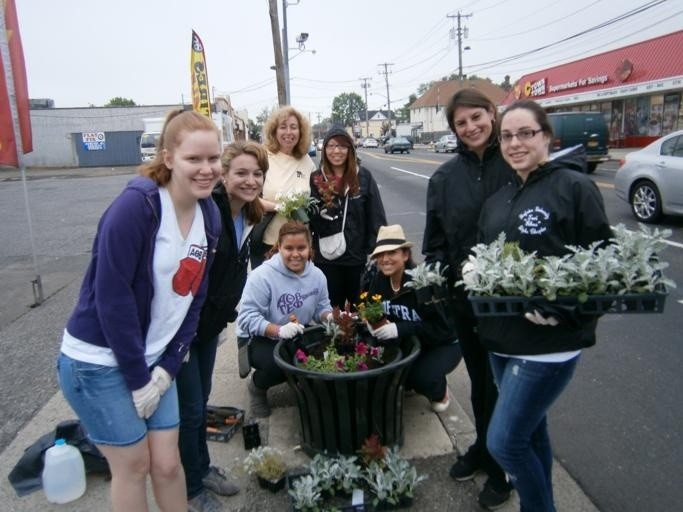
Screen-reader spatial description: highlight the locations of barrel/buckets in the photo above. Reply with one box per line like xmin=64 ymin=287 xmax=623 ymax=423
xmin=40 ymin=438 xmax=88 ymax=506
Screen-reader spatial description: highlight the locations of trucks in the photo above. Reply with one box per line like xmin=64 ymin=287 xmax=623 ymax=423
xmin=136 ymin=117 xmax=165 ymax=168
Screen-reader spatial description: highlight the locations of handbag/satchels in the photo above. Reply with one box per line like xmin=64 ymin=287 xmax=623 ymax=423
xmin=238 ymin=343 xmax=252 ymax=379
xmin=318 ymin=230 xmax=347 ymax=261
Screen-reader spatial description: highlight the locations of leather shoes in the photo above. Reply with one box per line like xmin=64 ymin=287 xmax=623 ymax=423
xmin=431 ymin=383 xmax=451 ymax=413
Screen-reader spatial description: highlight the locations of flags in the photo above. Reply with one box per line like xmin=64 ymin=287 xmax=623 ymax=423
xmin=0 ymin=0 xmax=37 ymax=171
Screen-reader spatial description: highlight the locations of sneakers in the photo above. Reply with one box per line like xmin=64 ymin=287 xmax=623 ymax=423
xmin=478 ymin=468 xmax=514 ymax=510
xmin=187 ymin=490 xmax=227 ymax=512
xmin=202 ymin=465 xmax=241 ymax=497
xmin=450 ymin=443 xmax=484 ymax=482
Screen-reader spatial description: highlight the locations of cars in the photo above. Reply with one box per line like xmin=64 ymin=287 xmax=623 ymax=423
xmin=611 ymin=129 xmax=682 ymax=227
xmin=383 ymin=137 xmax=411 ymax=154
xmin=434 ymin=135 xmax=458 ymax=155
xmin=308 ymin=136 xmax=379 ymax=156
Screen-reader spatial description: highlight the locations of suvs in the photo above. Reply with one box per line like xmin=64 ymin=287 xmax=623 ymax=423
xmin=542 ymin=110 xmax=611 ymax=176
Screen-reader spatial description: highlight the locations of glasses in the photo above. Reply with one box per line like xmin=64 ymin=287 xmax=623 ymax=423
xmin=325 ymin=143 xmax=348 ymax=151
xmin=497 ymin=128 xmax=545 ymax=144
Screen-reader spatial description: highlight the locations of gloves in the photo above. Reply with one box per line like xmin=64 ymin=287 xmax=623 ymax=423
xmin=374 ymin=321 xmax=399 ymax=341
xmin=326 ymin=309 xmax=359 ymax=323
xmin=524 ymin=310 xmax=559 ymax=326
xmin=277 ymin=321 xmax=306 ymax=339
xmin=131 ymin=366 xmax=172 ymax=419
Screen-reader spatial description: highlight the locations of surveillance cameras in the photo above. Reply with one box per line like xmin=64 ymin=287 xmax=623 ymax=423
xmin=296 ymin=32 xmax=309 ymax=43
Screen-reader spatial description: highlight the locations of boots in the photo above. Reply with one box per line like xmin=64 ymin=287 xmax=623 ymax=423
xmin=247 ymin=371 xmax=272 ymax=418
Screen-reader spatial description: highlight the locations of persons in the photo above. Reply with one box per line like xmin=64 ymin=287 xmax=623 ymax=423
xmin=357 ymin=221 xmax=463 ymax=414
xmin=52 ymin=105 xmax=225 ymax=511
xmin=172 ymin=137 xmax=269 ymax=512
xmin=419 ymin=86 xmax=519 ymax=511
xmin=449 ymin=98 xmax=617 ymax=511
xmin=306 ymin=120 xmax=389 ymax=312
xmin=232 ymin=222 xmax=358 ymax=421
xmin=246 ymin=104 xmax=319 ymax=275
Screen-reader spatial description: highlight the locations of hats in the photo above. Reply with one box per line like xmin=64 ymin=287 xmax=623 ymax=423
xmin=368 ymin=224 xmax=417 ymax=260
